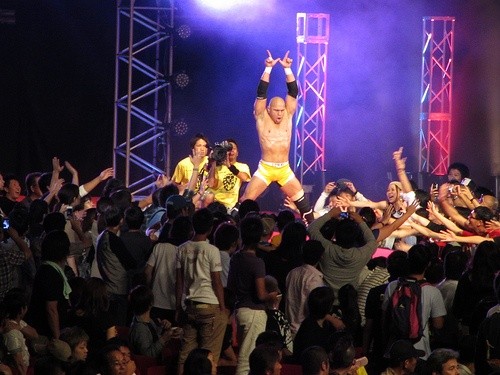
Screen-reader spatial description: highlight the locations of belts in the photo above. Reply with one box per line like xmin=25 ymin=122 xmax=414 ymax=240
xmin=185 ymin=300 xmax=204 ymax=306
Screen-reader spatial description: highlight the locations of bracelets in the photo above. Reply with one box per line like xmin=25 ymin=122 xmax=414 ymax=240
xmin=265 ymin=67 xmax=272 ymax=73
xmin=284 ymin=68 xmax=291 ymax=75
xmin=229 ymin=165 xmax=239 ymax=175
xmin=193 ymin=168 xmax=198 ymax=171
xmin=379 ymin=222 xmax=384 ymax=227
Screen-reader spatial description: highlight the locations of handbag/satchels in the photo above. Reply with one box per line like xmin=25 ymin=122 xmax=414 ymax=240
xmin=268 ymin=310 xmax=294 ymax=355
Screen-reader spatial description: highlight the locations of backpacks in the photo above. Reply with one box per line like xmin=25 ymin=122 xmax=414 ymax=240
xmin=387 ymin=277 xmax=432 ymax=345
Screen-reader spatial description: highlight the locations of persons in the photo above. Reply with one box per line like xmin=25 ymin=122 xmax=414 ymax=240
xmin=172 ymin=135 xmax=251 ymax=209
xmin=0 ymin=146 xmax=500 ymax=375
xmin=231 ymin=50 xmax=315 ymax=227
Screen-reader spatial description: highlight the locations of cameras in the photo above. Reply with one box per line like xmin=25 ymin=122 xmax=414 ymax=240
xmin=337 ymin=212 xmax=349 ymax=220
xmin=461 ymin=178 xmax=471 ymax=185
xmin=448 ymin=187 xmax=457 ymax=199
xmin=206 ymin=142 xmax=233 ymax=165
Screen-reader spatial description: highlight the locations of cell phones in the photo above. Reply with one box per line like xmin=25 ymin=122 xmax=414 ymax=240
xmin=3 ymin=218 xmax=9 ymax=229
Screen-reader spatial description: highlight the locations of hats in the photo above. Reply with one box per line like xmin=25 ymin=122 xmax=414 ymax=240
xmin=389 ymin=340 xmax=426 ymax=361
xmin=34 ymin=338 xmax=72 ymax=361
xmin=165 ymin=195 xmax=191 ymax=210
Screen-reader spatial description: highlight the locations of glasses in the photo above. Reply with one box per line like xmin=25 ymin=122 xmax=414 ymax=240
xmin=479 ymin=193 xmax=488 ymax=207
